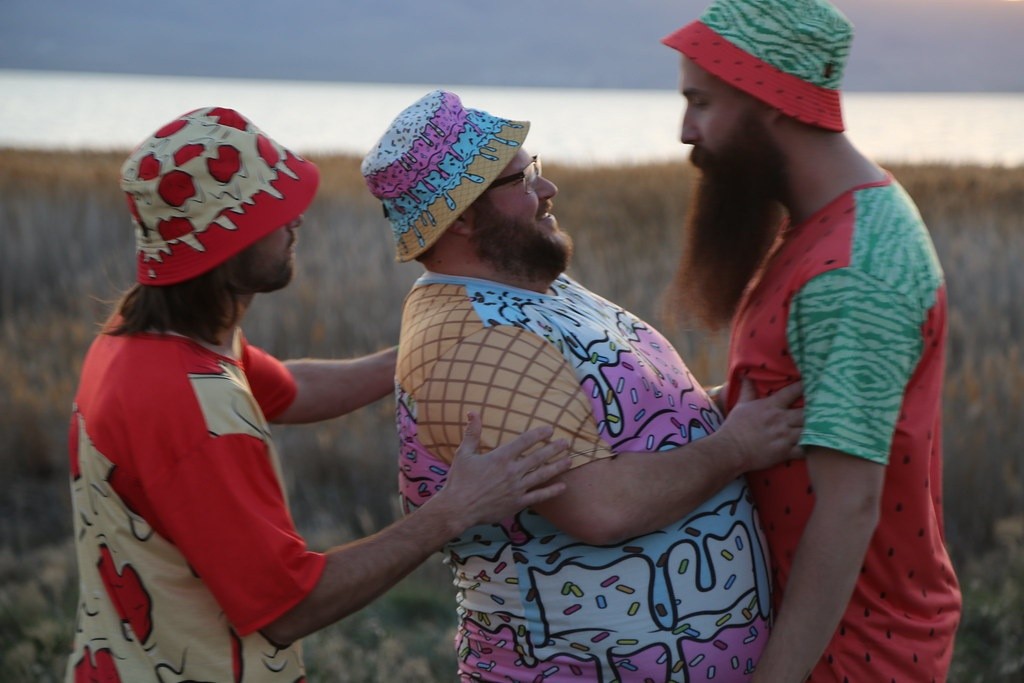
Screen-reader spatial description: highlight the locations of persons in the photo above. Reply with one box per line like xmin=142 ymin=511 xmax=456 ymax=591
xmin=63 ymin=104 xmax=575 ymax=683
xmin=660 ymin=0 xmax=963 ymax=683
xmin=362 ymin=88 xmax=810 ymax=683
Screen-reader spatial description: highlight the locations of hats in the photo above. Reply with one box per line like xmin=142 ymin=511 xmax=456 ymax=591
xmin=121 ymin=106 xmax=320 ymax=285
xmin=661 ymin=0 xmax=856 ymax=132
xmin=361 ymin=91 xmax=530 ymax=264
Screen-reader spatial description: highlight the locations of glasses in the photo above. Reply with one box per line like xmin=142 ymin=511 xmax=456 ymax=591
xmin=488 ymin=155 xmax=542 ymax=194
